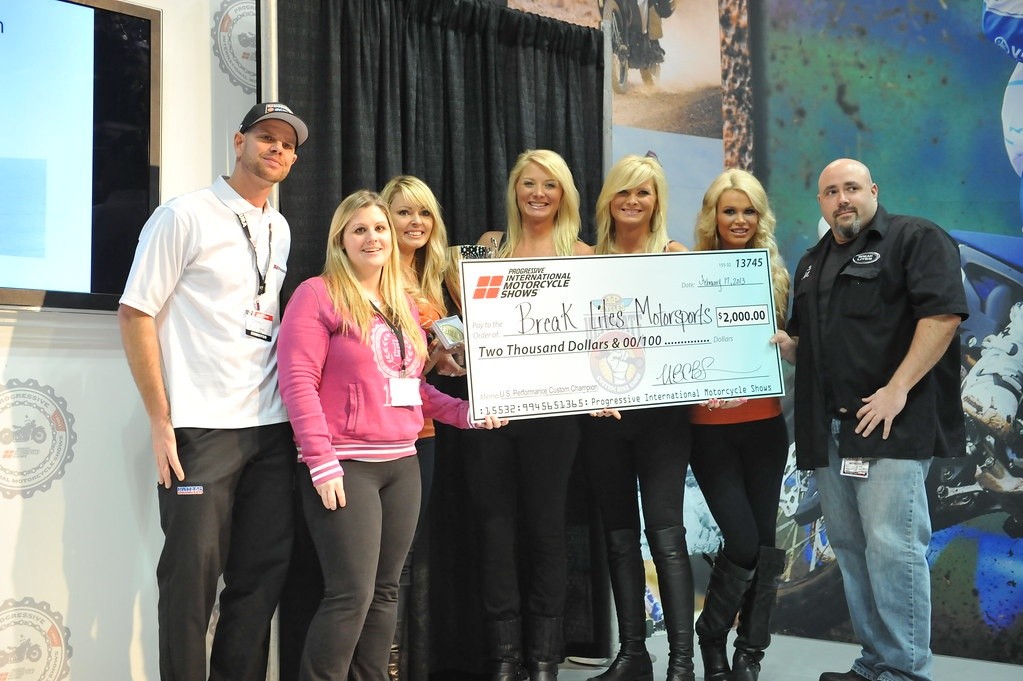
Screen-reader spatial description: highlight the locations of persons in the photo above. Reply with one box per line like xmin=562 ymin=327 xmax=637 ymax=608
xmin=276 ymin=189 xmax=508 ymax=681
xmin=689 ymin=169 xmax=790 ymax=681
xmin=584 ymin=155 xmax=696 ymax=681
xmin=473 ymin=150 xmax=622 ymax=680
xmin=768 ymin=158 xmax=972 ymax=681
xmin=117 ymin=101 xmax=309 ymax=681
xmin=378 ymin=175 xmax=467 ymax=681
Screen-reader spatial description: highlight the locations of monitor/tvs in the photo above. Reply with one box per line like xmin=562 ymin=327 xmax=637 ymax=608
xmin=0 ymin=0 xmax=164 ymax=312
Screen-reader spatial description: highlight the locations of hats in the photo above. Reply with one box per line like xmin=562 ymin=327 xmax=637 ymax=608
xmin=239 ymin=102 xmax=310 ymax=148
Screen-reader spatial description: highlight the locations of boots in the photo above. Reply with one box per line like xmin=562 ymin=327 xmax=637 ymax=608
xmin=733 ymin=545 xmax=787 ymax=681
xmin=587 ymin=529 xmax=653 ymax=681
xmin=526 ymin=611 xmax=563 ymax=681
xmin=645 ymin=526 xmax=695 ymax=681
xmin=485 ymin=614 xmax=530 ymax=681
xmin=695 ymin=538 xmax=758 ymax=681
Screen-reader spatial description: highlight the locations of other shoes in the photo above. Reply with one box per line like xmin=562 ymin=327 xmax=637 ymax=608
xmin=388 ymin=644 xmax=402 ymax=681
xmin=820 ymin=670 xmax=872 ymax=681
